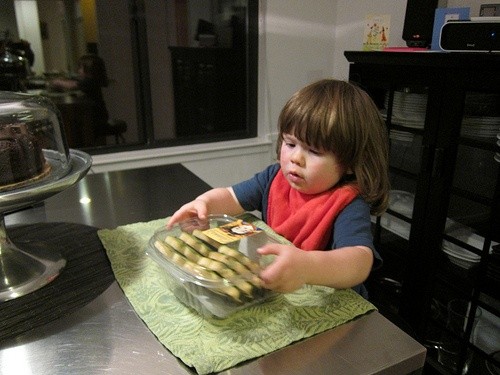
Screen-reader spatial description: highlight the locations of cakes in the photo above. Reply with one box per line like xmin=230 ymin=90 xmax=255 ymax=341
xmin=0 ymin=123 xmax=46 ymax=186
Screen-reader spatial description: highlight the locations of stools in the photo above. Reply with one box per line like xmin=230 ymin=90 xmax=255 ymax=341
xmin=98 ymin=119 xmax=128 ymax=144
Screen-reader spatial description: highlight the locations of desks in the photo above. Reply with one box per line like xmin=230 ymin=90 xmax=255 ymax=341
xmin=0 ymin=163 xmax=427 ymax=375
xmin=31 ymin=79 xmax=97 ymax=150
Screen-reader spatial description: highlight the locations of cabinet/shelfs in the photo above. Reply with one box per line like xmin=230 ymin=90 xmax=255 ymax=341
xmin=344 ymin=51 xmax=500 ymax=375
xmin=168 ymin=45 xmax=248 ymax=137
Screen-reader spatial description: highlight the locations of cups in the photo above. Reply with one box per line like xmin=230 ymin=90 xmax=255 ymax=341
xmin=437 ymin=300 xmax=483 ymax=375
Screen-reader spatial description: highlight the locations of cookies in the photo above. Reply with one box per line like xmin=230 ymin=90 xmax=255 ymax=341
xmin=153 ymin=229 xmax=268 ymax=304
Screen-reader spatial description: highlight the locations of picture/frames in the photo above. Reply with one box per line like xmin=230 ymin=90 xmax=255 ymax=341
xmin=431 ymin=8 xmax=471 ymax=51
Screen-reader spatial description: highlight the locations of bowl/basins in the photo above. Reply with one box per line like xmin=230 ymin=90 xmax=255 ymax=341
xmin=441 ymin=228 xmax=499 ymax=269
xmin=147 ymin=214 xmax=281 ymax=320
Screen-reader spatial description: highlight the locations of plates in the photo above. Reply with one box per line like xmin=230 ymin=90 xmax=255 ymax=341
xmin=460 ymin=116 xmax=500 ymax=163
xmin=379 ymin=90 xmax=428 ymax=131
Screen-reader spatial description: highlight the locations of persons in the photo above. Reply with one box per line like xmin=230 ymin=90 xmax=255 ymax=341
xmin=50 ymin=55 xmax=108 ymax=146
xmin=168 ymin=78 xmax=390 ymax=294
